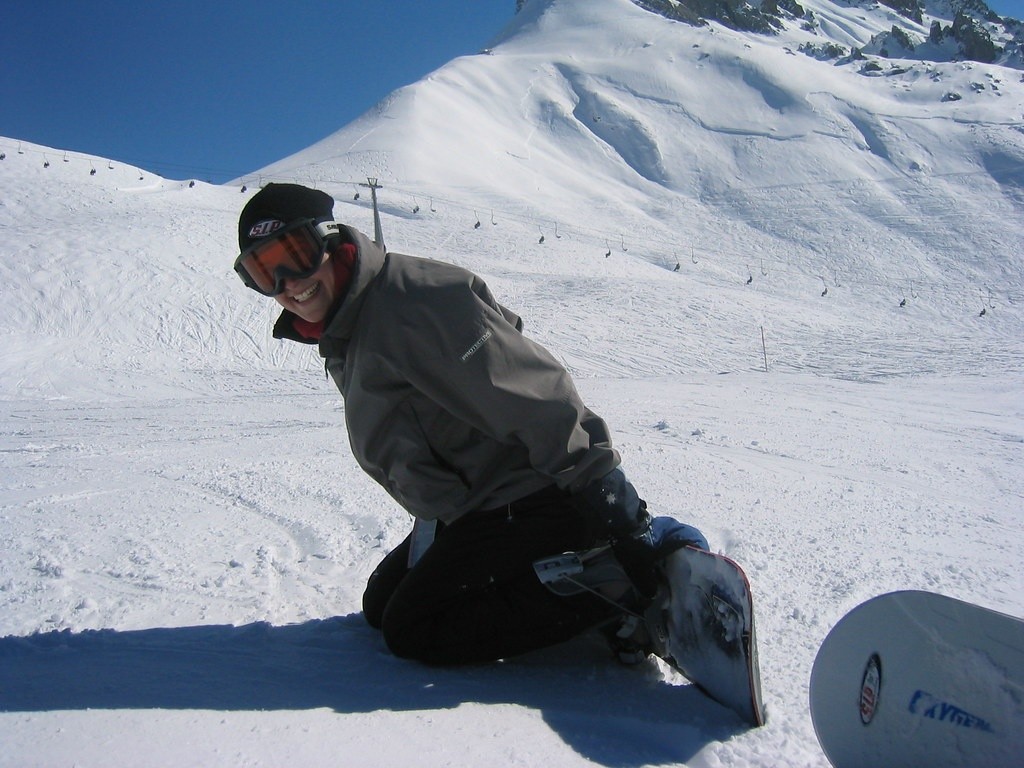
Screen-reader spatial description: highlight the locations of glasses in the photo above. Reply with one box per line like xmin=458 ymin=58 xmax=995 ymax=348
xmin=234 ymin=216 xmax=340 ymax=297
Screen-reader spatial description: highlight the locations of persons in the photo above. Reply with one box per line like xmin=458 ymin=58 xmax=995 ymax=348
xmin=235 ymin=183 xmax=710 ymax=670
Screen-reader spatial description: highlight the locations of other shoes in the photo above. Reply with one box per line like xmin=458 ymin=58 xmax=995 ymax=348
xmin=585 ymin=548 xmax=652 ymax=666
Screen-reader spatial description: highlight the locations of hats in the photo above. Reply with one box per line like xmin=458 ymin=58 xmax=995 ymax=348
xmin=238 ymin=182 xmax=334 ymax=252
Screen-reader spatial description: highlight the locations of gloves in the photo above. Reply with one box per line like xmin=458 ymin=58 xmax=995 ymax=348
xmin=585 ymin=475 xmax=652 ymax=585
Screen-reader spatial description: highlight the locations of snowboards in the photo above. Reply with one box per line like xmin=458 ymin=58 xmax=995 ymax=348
xmin=651 ymin=542 xmax=766 ymax=730
xmin=807 ymin=589 xmax=1024 ymax=768
